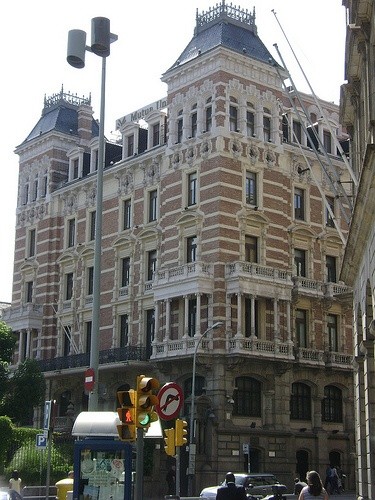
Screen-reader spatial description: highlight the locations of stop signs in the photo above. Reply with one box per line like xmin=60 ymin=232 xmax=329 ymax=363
xmin=84 ymin=368 xmax=95 ymax=391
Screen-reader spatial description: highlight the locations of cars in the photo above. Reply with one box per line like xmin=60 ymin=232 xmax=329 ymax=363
xmin=223 ymin=470 xmax=289 ymax=497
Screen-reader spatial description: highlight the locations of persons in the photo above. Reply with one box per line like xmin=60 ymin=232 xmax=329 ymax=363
xmin=9 ymin=469 xmax=23 ymax=496
xmin=324 ymin=463 xmax=336 ymax=495
xmin=335 ymin=463 xmax=345 ymax=495
xmin=216 ymin=472 xmax=249 ymax=500
xmin=294 ymin=477 xmax=308 ymax=495
xmin=298 ymin=471 xmax=329 ymax=500
xmin=66 ymin=469 xmax=74 ymax=478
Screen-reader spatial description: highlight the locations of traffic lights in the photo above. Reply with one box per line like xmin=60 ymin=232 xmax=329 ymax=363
xmin=117 ymin=390 xmax=136 ymax=442
xmin=163 ymin=429 xmax=175 ymax=457
xmin=176 ymin=416 xmax=187 ymax=447
xmin=135 ymin=374 xmax=159 ymax=429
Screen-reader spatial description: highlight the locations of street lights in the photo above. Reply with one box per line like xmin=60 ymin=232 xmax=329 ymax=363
xmin=187 ymin=322 xmax=224 ymax=497
